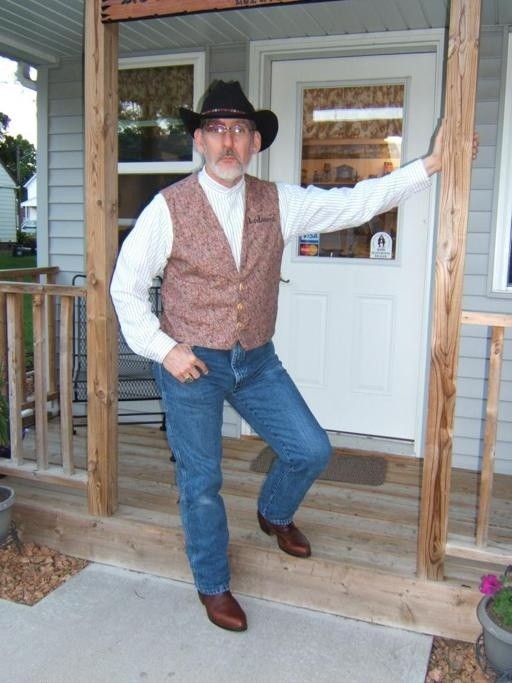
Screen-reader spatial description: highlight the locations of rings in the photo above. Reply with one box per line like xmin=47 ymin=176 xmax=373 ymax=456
xmin=185 ymin=376 xmax=193 ymax=383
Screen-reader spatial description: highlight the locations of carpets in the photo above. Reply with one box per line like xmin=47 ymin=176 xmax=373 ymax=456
xmin=249 ymin=436 xmax=388 ymax=488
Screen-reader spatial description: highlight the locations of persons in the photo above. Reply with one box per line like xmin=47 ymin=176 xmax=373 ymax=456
xmin=108 ymin=79 xmax=479 ymax=632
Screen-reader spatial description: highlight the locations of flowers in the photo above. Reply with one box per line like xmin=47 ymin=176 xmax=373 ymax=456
xmin=476 ymin=562 xmax=512 ymax=630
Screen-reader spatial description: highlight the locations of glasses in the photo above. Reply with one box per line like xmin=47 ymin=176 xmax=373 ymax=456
xmin=200 ymin=121 xmax=260 ymax=139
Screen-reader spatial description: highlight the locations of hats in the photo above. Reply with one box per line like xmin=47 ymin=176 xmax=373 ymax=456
xmin=179 ymin=78 xmax=278 ymax=151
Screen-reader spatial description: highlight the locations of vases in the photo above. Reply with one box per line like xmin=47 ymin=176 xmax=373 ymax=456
xmin=470 ymin=580 xmax=511 ymax=677
xmin=0 ymin=484 xmax=19 ymax=546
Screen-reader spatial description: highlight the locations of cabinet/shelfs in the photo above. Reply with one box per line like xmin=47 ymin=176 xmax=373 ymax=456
xmin=300 ymin=132 xmax=403 ymax=189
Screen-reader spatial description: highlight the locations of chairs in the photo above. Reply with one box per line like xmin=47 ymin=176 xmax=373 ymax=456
xmin=55 ymin=270 xmax=177 ymax=465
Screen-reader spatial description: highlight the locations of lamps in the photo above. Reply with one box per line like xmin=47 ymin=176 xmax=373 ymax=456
xmin=310 ymin=102 xmax=403 ymax=124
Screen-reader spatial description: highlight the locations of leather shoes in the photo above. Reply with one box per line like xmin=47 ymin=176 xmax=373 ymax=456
xmin=257 ymin=510 xmax=312 ymax=557
xmin=198 ymin=588 xmax=247 ymax=632
